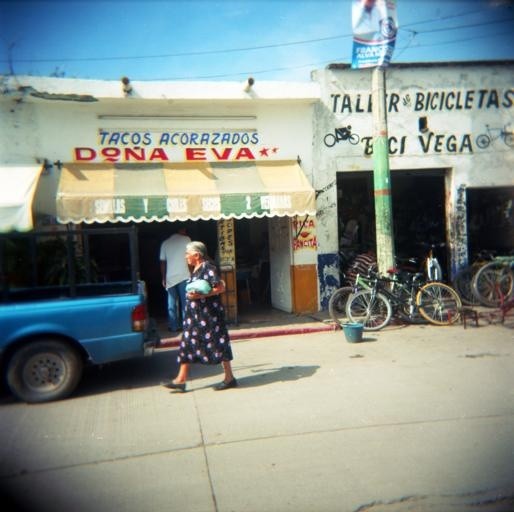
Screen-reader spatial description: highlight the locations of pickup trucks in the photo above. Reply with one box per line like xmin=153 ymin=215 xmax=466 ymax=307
xmin=0 ymin=282 xmax=152 ymax=400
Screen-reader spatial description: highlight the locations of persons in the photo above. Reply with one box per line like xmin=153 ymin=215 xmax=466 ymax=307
xmin=162 ymin=240 xmax=239 ymax=392
xmin=342 ymin=244 xmax=378 ymax=286
xmin=158 ymin=227 xmax=193 ymax=333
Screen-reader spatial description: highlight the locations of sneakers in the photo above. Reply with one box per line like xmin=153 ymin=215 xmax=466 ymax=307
xmin=163 ymin=382 xmax=186 ymax=392
xmin=214 ymin=378 xmax=237 ymax=390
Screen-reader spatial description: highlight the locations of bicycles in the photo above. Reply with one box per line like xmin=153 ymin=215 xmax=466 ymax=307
xmin=350 ymin=264 xmax=462 ymax=329
xmin=472 ymin=256 xmax=513 ymax=305
xmin=327 ymin=269 xmax=435 ymax=326
xmin=455 ymin=251 xmax=493 ymax=305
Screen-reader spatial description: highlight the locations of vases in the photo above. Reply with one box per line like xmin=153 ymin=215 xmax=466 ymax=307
xmin=340 ymin=322 xmax=364 ymax=343
